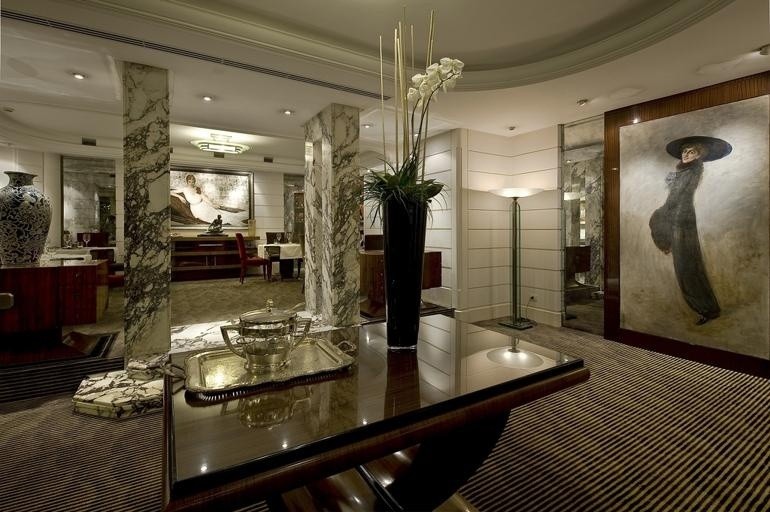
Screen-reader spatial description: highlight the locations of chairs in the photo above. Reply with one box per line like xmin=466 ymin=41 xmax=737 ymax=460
xmin=236 ymin=233 xmax=270 ymax=284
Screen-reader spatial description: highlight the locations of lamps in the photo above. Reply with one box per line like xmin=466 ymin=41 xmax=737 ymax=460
xmin=192 ymin=135 xmax=250 ymax=155
xmin=493 ymin=188 xmax=545 ymax=330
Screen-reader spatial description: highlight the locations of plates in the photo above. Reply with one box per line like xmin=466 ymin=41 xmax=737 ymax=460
xmin=165 ymin=337 xmax=357 ymax=392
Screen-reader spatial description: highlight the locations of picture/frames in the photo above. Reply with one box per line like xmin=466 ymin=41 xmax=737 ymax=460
xmin=60 ymin=156 xmax=255 ymax=246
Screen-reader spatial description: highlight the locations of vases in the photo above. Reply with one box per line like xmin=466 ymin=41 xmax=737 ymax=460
xmin=380 ymin=203 xmax=427 ymax=351
xmin=0 ymin=172 xmax=52 ymax=266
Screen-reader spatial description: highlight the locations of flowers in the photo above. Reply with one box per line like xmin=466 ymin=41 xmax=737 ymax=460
xmin=329 ymin=10 xmax=464 ymax=250
